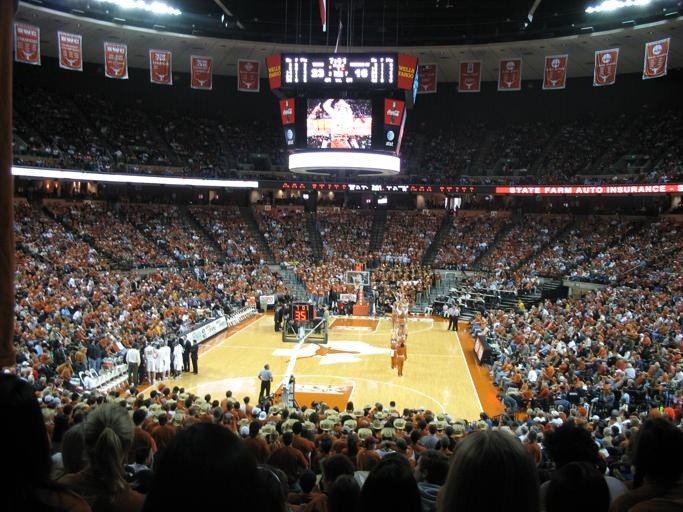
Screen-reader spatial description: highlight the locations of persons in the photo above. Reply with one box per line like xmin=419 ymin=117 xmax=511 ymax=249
xmin=0 ymin=87 xmax=683 ymax=512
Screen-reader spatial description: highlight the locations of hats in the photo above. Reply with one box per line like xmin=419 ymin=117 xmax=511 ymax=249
xmin=478 ymin=420 xmax=488 ymax=432
xmin=252 ymin=405 xmax=464 ymax=442
xmin=150 ymin=397 xmax=211 ymax=425
